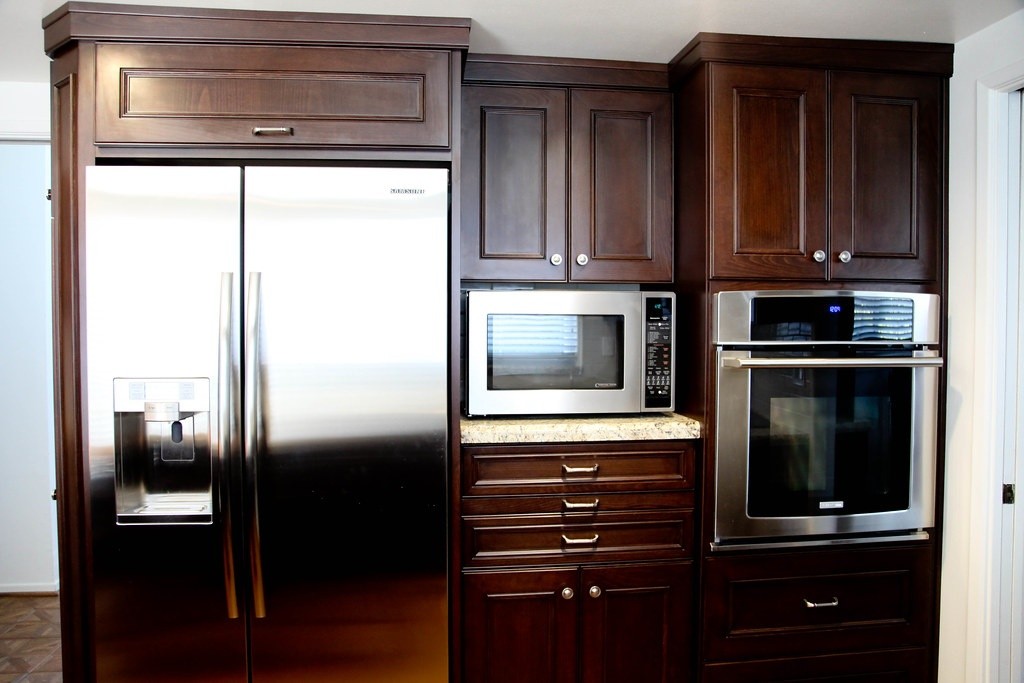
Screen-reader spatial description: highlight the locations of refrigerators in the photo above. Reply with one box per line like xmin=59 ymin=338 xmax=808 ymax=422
xmin=76 ymin=165 xmax=450 ymax=683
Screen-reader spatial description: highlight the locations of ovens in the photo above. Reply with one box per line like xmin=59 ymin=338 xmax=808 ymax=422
xmin=710 ymin=289 xmax=944 ymax=553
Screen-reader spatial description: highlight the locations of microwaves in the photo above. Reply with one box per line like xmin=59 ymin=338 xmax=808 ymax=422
xmin=465 ymin=289 xmax=676 ymax=418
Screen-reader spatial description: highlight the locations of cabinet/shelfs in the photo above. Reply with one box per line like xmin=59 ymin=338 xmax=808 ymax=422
xmin=75 ymin=36 xmax=464 ymax=160
xmin=666 ymin=57 xmax=950 ymax=285
xmin=454 ymin=439 xmax=940 ymax=683
xmin=463 ymin=50 xmax=676 ymax=284
